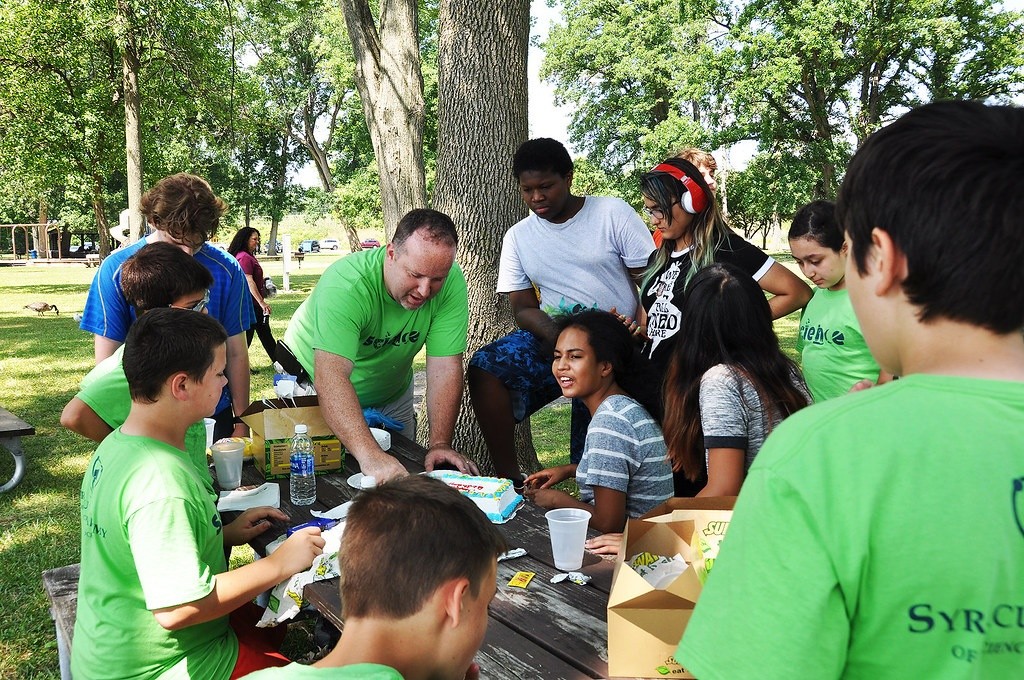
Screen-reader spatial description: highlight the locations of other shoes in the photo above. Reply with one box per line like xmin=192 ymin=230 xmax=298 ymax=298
xmin=314 ymin=614 xmax=340 ymax=649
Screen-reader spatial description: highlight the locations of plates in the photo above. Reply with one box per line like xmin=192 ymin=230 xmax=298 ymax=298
xmin=345 ymin=427 xmax=392 ymax=454
xmin=347 ymin=472 xmax=366 ymax=490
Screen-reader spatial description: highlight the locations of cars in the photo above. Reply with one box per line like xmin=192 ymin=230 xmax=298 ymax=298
xmin=264 ymin=240 xmax=283 ymax=253
xmin=70 ymin=242 xmax=100 ymax=252
xmin=207 ymin=242 xmax=229 ymax=251
xmin=360 ymin=238 xmax=380 ymax=248
xmin=299 ymin=240 xmax=320 ymax=252
xmin=251 ymin=242 xmax=261 ymax=255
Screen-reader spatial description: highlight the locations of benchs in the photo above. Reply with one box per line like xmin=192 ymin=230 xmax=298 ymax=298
xmin=42 ymin=563 xmax=81 ymax=680
xmin=83 ymin=253 xmax=100 ymax=268
xmin=0 ymin=405 xmax=35 ymax=494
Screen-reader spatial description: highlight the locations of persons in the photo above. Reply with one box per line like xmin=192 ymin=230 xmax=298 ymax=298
xmin=71 ymin=308 xmax=326 ymax=680
xmin=609 ymin=158 xmax=815 ymax=497
xmin=236 ymin=475 xmax=510 ymax=680
xmin=674 ymin=102 xmax=1024 ymax=680
xmin=276 ymin=208 xmax=481 ymax=487
xmin=788 ymin=201 xmax=898 ymax=402
xmin=522 ymin=309 xmax=674 ymax=533
xmin=79 ymin=173 xmax=251 ymax=569
xmin=228 ymin=226 xmax=277 ymax=373
xmin=635 ymin=148 xmax=718 ymax=338
xmin=585 ymin=263 xmax=812 ymax=555
xmin=467 ymin=139 xmax=658 ymax=496
xmin=59 ymin=240 xmax=217 ymax=501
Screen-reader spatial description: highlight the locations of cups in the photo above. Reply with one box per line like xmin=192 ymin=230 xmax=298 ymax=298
xmin=204 ymin=418 xmax=216 ymax=454
xmin=544 ymin=507 xmax=592 ymax=570
xmin=210 ymin=441 xmax=245 ymax=489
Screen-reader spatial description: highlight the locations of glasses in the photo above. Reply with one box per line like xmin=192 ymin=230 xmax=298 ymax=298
xmin=645 ymin=200 xmax=681 ymax=219
xmin=170 ymin=288 xmax=210 ymax=312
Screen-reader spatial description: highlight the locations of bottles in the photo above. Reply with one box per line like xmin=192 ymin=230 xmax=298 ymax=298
xmin=289 ymin=424 xmax=317 ymax=506
xmin=262 ymin=309 xmax=269 ymax=326
xmin=357 ymin=476 xmax=376 ymax=496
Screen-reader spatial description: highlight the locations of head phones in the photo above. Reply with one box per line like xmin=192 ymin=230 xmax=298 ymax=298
xmin=650 ymin=164 xmax=708 ymax=214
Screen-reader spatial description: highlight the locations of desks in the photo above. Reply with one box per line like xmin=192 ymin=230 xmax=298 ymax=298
xmin=206 ymin=427 xmax=617 ymax=680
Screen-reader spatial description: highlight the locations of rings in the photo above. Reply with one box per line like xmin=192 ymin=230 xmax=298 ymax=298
xmin=466 ymin=460 xmax=470 ymax=463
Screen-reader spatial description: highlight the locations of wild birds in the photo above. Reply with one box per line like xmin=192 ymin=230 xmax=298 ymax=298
xmin=23 ymin=300 xmax=60 ymax=317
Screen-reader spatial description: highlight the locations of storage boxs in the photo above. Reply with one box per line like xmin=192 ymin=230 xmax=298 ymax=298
xmin=239 ymin=395 xmax=345 ymax=481
xmin=607 ymin=495 xmax=738 ymax=679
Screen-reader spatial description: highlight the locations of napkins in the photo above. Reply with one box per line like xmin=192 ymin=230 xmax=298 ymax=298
xmin=217 ymin=482 xmax=280 ymax=512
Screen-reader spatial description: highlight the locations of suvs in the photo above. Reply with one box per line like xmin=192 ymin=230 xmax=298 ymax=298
xmin=319 ymin=239 xmax=340 ymax=250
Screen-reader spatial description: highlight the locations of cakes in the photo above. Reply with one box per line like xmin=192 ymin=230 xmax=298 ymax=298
xmin=423 ymin=473 xmax=523 ymax=523
